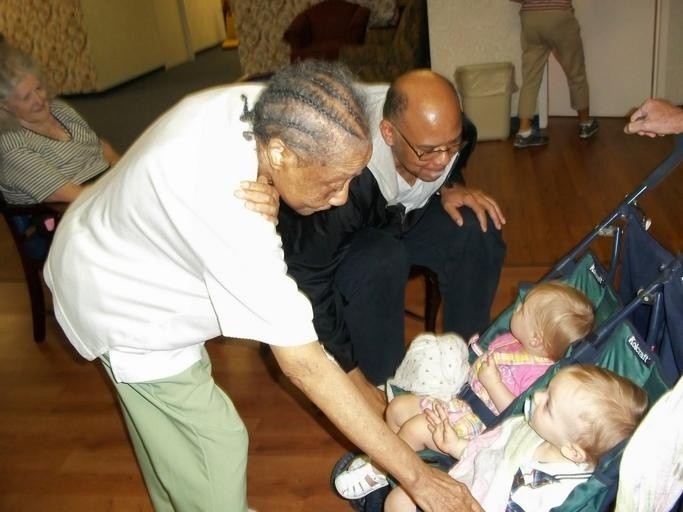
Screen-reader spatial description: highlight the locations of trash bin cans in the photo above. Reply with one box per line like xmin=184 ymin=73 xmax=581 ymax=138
xmin=457 ymin=63 xmax=514 ymax=143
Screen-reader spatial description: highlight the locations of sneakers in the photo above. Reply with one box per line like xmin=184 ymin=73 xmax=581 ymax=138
xmin=578 ymin=115 xmax=600 ymax=138
xmin=513 ymin=128 xmax=550 ymax=148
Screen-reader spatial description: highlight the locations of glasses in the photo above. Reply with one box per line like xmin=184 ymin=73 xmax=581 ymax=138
xmin=388 ymin=119 xmax=469 ymax=162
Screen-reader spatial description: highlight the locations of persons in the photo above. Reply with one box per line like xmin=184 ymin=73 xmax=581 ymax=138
xmin=384 ymin=362 xmax=651 ymax=511
xmin=43 ymin=63 xmax=484 ymax=512
xmin=272 ymin=66 xmax=506 ymax=402
xmin=624 ymin=98 xmax=683 ymax=138
xmin=512 ymin=0 xmax=598 ymax=147
xmin=333 ymin=280 xmax=594 ymax=500
xmin=284 ymin=0 xmax=397 ymax=67
xmin=0 ymin=41 xmax=120 ymax=230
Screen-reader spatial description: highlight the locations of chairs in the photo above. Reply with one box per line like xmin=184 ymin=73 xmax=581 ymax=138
xmin=0 ymin=194 xmax=77 ymax=344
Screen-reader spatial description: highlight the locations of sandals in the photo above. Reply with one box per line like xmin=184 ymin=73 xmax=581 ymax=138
xmin=334 ymin=463 xmax=392 ymax=501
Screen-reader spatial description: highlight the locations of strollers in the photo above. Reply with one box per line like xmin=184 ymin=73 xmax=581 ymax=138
xmin=330 ymin=130 xmax=681 ymax=507
